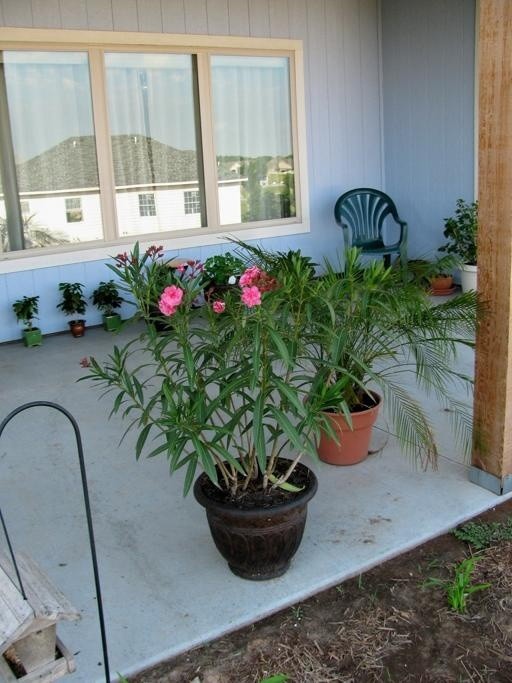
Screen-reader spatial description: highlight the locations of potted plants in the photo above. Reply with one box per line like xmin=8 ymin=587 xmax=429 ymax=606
xmin=12 ymin=296 xmax=41 ymax=347
xmin=56 ymin=283 xmax=87 ymax=338
xmin=89 ymin=280 xmax=121 ymax=332
xmin=426 ymin=254 xmax=464 ymax=295
xmin=218 ymin=233 xmax=490 ymax=474
xmin=437 ymin=199 xmax=478 ymax=292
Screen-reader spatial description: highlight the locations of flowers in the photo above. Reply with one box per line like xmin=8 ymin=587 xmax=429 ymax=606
xmin=79 ymin=268 xmax=354 ymax=489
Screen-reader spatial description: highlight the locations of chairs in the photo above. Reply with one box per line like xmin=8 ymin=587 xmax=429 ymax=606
xmin=334 ymin=187 xmax=407 ymax=282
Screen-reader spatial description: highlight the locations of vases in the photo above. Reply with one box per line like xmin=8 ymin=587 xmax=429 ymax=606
xmin=192 ymin=455 xmax=318 ymax=580
